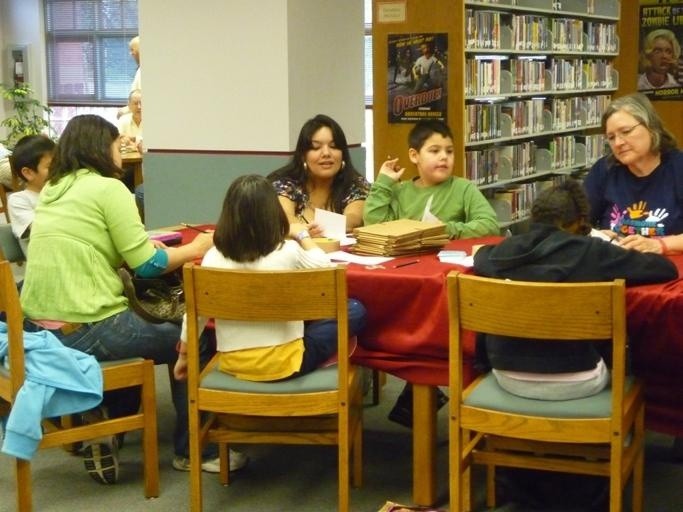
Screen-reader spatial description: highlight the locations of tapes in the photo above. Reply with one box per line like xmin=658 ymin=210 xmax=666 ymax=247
xmin=313 ymin=238 xmax=340 ymax=253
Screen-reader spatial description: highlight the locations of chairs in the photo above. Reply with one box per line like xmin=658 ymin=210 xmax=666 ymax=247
xmin=445 ymin=267 xmax=644 ymax=512
xmin=0 ymin=256 xmax=158 ymax=512
xmin=0 ymin=184 xmax=28 ymax=287
xmin=180 ymin=260 xmax=366 ymax=512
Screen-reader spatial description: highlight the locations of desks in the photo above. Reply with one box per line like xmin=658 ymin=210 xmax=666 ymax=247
xmin=144 ymin=220 xmax=680 ymax=509
xmin=118 ymin=149 xmax=141 ymax=193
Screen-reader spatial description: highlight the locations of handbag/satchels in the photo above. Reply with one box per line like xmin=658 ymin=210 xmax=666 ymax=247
xmin=125 ymin=264 xmax=187 ymax=326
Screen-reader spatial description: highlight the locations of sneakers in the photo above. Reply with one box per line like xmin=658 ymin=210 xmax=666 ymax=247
xmin=76 ymin=406 xmax=123 ymax=486
xmin=174 ymin=442 xmax=246 ymax=476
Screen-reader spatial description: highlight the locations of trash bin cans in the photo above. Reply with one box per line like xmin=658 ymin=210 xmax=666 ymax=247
xmin=135 ymin=183 xmax=144 ymax=224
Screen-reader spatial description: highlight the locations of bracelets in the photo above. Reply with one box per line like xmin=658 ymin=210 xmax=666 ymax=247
xmin=655 ymin=237 xmax=668 ymax=255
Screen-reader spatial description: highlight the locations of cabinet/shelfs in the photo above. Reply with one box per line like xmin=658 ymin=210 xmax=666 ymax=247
xmin=371 ymin=0 xmax=620 ymax=245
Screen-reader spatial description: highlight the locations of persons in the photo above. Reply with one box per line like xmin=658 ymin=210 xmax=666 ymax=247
xmin=111 ymin=88 xmax=143 ymax=155
xmin=2 ymin=135 xmax=58 ymax=269
xmin=467 ymin=177 xmax=679 ymax=403
xmin=577 ymin=90 xmax=682 ymax=260
xmin=133 ymin=139 xmax=142 ymax=212
xmin=166 ymin=166 xmax=369 ymax=384
xmin=360 ymin=115 xmax=501 ymax=246
xmin=407 ymin=38 xmax=443 ymax=94
xmin=16 ymin=111 xmax=251 ymax=487
xmin=113 ymin=36 xmax=140 ymax=119
xmin=264 ymin=109 xmax=372 ymax=238
xmin=631 ymin=28 xmax=679 ymax=95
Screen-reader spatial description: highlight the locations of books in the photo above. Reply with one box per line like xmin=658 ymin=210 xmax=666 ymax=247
xmin=458 ymin=7 xmax=618 ymax=225
xmin=348 ymin=216 xmax=449 ymax=258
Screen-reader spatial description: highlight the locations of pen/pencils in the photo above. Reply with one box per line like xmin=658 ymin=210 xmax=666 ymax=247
xmin=180 ymin=223 xmax=209 ymax=233
xmin=394 ymin=259 xmax=419 ymax=268
xmin=610 ymin=232 xmax=621 ymax=242
xmin=301 ymin=216 xmax=312 ymax=230
xmin=387 ymin=155 xmax=401 ymax=182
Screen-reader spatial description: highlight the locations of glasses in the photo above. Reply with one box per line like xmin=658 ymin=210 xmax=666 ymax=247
xmin=606 ymin=122 xmax=645 ymax=144
